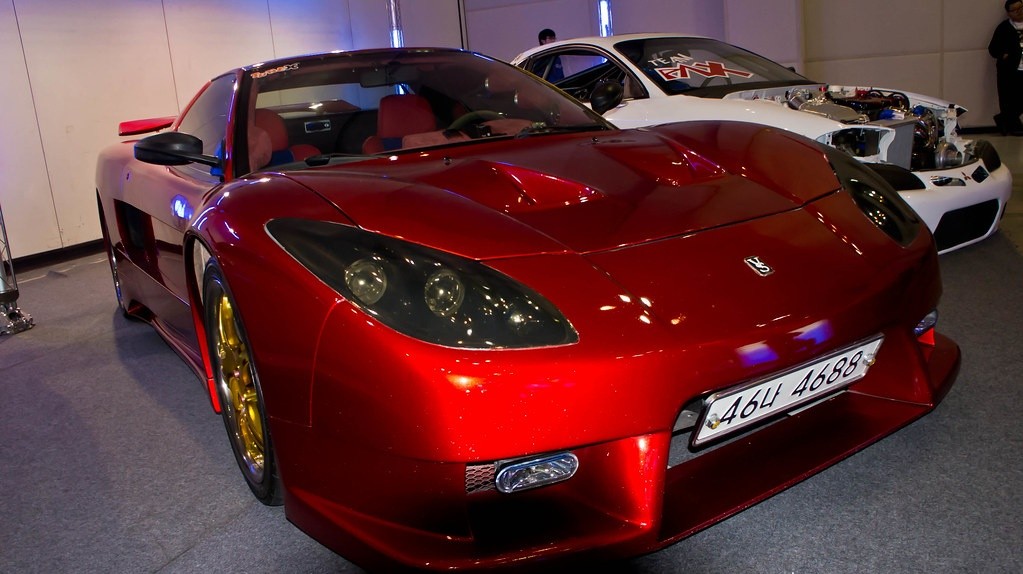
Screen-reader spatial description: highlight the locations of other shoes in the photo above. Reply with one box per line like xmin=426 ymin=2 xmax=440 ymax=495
xmin=994 ymin=112 xmax=1008 ymax=136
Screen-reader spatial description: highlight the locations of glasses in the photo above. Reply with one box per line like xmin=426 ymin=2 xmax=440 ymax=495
xmin=546 ymin=37 xmax=557 ymax=39
xmin=1008 ymin=6 xmax=1023 ymax=12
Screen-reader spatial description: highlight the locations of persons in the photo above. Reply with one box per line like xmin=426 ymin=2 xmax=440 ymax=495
xmin=529 ymin=28 xmax=565 ymax=84
xmin=988 ymin=0 xmax=1023 ymax=137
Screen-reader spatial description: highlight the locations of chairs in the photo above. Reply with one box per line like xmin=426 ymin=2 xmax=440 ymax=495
xmin=363 ymin=92 xmax=436 ymax=159
xmin=250 ymin=107 xmax=320 ymax=170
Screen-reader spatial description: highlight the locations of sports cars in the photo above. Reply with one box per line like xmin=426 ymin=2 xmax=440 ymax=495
xmin=510 ymin=34 xmax=1013 ymax=256
xmin=96 ymin=47 xmax=962 ymax=574
